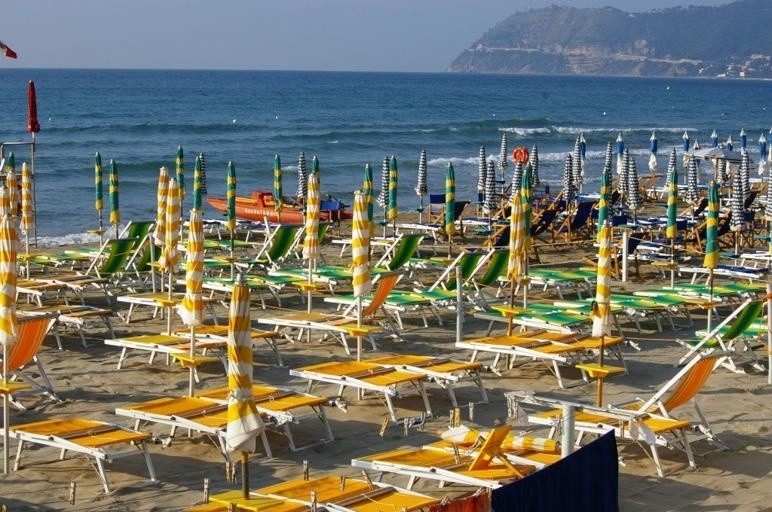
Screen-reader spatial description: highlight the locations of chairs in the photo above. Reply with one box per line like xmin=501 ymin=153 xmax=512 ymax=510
xmin=0 ymin=173 xmax=771 ymax=512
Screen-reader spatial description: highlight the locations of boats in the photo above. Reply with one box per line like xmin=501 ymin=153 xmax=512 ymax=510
xmin=207 ymin=191 xmax=353 ymax=224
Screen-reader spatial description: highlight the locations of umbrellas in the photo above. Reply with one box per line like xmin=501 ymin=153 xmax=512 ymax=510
xmin=28 ymin=78 xmax=39 ymax=152
xmin=0 ymin=40 xmax=17 ymax=58
